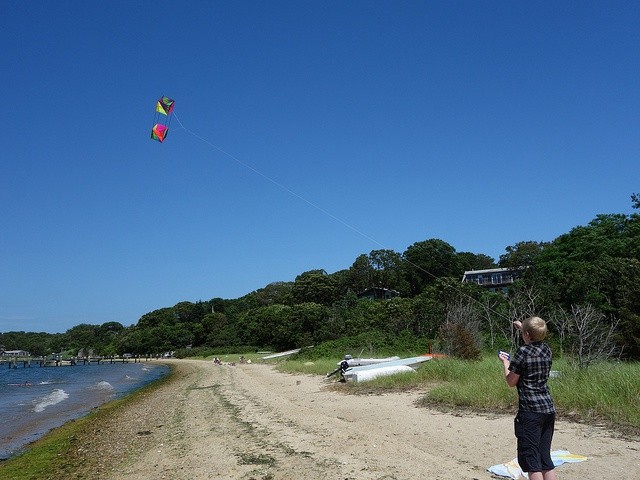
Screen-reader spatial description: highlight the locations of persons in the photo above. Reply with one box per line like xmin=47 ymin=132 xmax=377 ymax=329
xmin=498 ymin=315 xmax=557 ymax=480
xmin=149 ymin=95 xmax=174 ymax=143
